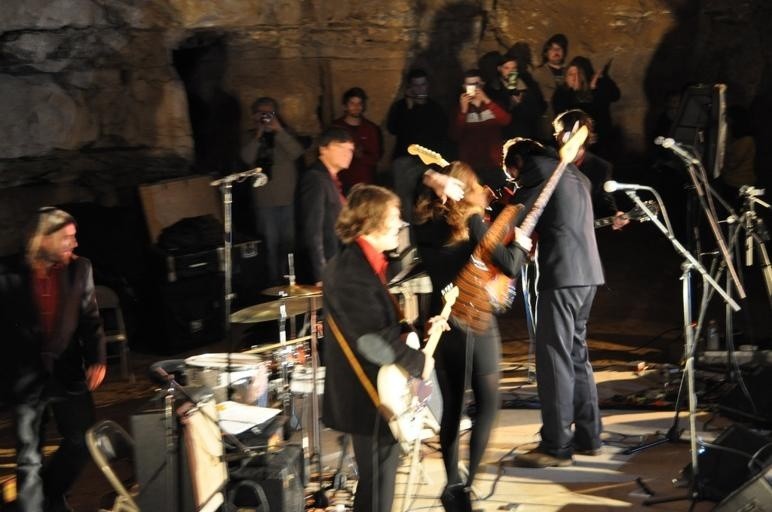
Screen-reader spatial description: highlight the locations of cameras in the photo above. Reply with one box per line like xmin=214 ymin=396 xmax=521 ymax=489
xmin=505 ymin=71 xmax=521 ymax=88
xmin=413 ymin=90 xmax=428 ymax=99
xmin=260 ymin=111 xmax=277 ymax=125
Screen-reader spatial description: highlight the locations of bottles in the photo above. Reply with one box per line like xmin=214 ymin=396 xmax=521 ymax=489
xmin=706 ymin=319 xmax=720 ymax=351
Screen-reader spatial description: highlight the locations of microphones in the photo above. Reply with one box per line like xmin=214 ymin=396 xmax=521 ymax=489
xmin=602 ymin=179 xmax=640 ymax=194
xmin=662 ymin=138 xmax=698 ymax=166
xmin=247 ymin=170 xmax=270 ymax=188
xmin=655 ymin=135 xmax=683 ymax=149
xmin=225 ymin=433 xmax=252 ymax=458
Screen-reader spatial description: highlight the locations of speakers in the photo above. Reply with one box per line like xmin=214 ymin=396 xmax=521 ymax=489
xmin=130 ymin=385 xmax=229 ymax=512
xmin=231 ymin=441 xmax=306 ymax=511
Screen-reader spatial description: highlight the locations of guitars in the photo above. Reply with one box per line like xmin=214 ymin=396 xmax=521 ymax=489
xmin=377 ymin=282 xmax=459 ymax=443
xmin=409 ymin=145 xmax=538 ymax=262
xmin=446 ymin=121 xmax=590 ymax=335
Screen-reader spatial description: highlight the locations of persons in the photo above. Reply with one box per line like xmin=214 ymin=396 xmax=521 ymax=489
xmin=321 ymin=182 xmax=451 ymax=512
xmin=0 ymin=206 xmax=107 ymax=512
xmin=241 ymin=33 xmax=631 ymax=288
xmin=411 ymin=161 xmax=534 ymax=512
xmin=719 ymin=105 xmax=756 ymax=202
xmin=655 ymin=89 xmax=680 ymax=138
xmin=483 ymin=137 xmax=606 ymax=467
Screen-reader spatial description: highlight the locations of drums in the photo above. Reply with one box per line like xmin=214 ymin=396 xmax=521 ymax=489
xmin=185 ymin=353 xmax=269 ymax=409
xmin=253 ymin=343 xmax=311 ymax=379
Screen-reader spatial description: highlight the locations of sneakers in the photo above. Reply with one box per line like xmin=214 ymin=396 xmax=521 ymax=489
xmin=568 ymin=448 xmax=602 ymax=455
xmin=513 ymin=447 xmax=571 ymax=467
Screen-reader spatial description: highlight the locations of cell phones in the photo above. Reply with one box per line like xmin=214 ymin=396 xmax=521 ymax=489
xmin=466 ymin=84 xmax=477 ymax=97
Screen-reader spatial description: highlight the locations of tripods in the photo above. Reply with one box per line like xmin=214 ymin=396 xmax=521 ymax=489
xmin=621 ymin=233 xmax=724 ymax=456
xmin=640 ymin=263 xmax=734 ymax=512
xmin=292 ymin=308 xmax=358 ymax=512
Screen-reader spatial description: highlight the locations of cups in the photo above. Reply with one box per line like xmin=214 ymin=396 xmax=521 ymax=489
xmin=507 ymin=71 xmax=519 ymax=90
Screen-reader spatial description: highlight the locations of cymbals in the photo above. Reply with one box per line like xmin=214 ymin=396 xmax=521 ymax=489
xmin=229 ymin=297 xmax=324 ymax=324
xmin=263 ymin=283 xmax=324 ymax=296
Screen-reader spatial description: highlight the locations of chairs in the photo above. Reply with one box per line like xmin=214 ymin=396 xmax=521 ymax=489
xmin=94 ymin=286 xmax=131 ymax=384
